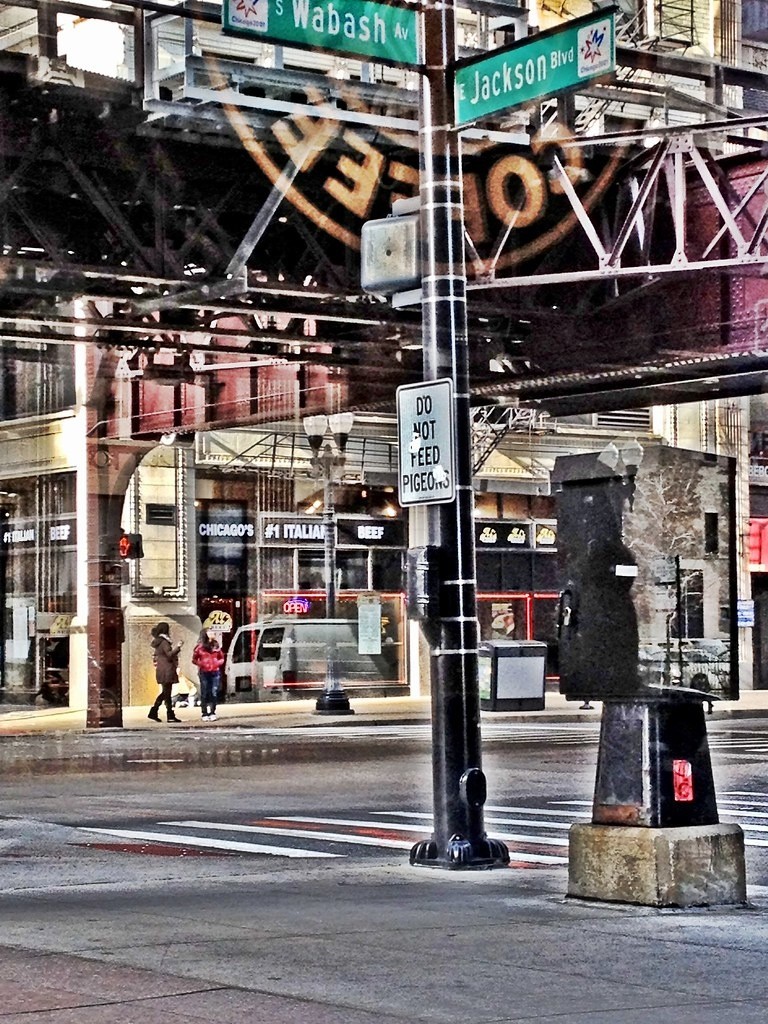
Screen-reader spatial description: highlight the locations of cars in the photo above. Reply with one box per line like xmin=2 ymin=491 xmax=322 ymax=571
xmin=23 ymin=635 xmax=70 ymax=703
xmin=639 ymin=638 xmax=730 ymax=692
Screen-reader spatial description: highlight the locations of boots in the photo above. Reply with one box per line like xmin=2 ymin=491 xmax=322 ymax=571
xmin=167 ymin=710 xmax=181 ymax=722
xmin=148 ymin=707 xmax=161 ymax=722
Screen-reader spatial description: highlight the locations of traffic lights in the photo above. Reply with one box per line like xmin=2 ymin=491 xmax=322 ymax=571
xmin=120 ymin=533 xmax=144 ymax=560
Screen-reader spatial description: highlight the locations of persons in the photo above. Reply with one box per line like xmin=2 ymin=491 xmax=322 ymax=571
xmin=147 ymin=622 xmax=183 ymax=722
xmin=191 ymin=627 xmax=224 ymax=722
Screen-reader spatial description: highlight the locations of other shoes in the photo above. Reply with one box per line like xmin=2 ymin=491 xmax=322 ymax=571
xmin=209 ymin=714 xmax=218 ymax=721
xmin=201 ymin=715 xmax=209 ymax=721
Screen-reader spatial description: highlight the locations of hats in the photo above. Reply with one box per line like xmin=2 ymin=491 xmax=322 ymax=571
xmin=151 ymin=622 xmax=169 ymax=638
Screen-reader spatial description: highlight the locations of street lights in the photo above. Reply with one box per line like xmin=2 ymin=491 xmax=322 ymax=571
xmin=303 ymin=412 xmax=355 ymax=716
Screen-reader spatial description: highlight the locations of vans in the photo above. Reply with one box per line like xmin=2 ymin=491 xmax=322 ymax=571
xmin=224 ymin=620 xmax=399 ymax=703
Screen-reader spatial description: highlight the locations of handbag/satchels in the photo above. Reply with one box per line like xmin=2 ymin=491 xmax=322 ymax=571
xmin=152 ymin=648 xmax=179 ymax=667
